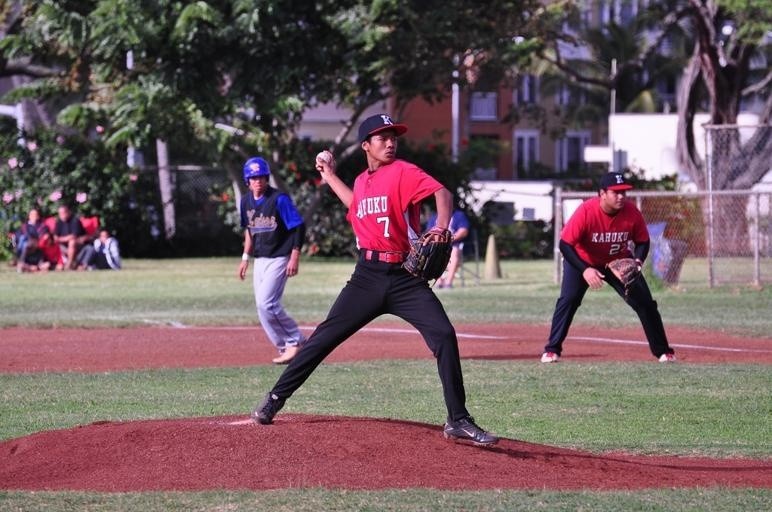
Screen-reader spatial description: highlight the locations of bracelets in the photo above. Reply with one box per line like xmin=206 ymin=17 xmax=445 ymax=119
xmin=241 ymin=251 xmax=252 ymax=263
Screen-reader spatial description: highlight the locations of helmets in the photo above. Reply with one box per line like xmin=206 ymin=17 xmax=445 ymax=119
xmin=243 ymin=157 xmax=270 ymax=186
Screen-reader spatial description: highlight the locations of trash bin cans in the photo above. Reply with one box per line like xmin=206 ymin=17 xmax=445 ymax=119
xmin=647 ymin=222 xmax=691 ymax=284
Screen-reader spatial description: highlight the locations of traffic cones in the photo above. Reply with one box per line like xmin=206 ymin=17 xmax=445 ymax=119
xmin=478 ymin=232 xmax=508 ymax=284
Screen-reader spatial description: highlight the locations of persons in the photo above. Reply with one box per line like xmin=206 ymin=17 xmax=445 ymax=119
xmin=251 ymin=114 xmax=501 ymax=448
xmin=539 ymin=171 xmax=677 ymax=364
xmin=237 ymin=156 xmax=309 ymax=365
xmin=422 ymin=195 xmax=471 ymax=290
xmin=9 ymin=204 xmax=123 ymax=274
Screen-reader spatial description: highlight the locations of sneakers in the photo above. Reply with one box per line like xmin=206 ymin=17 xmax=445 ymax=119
xmin=255 ymin=391 xmax=285 ymax=425
xmin=657 ymin=352 xmax=677 ymax=363
xmin=435 ymin=280 xmax=453 ymax=290
xmin=444 ymin=416 xmax=499 ymax=449
xmin=272 ymin=340 xmax=305 ymax=364
xmin=540 ymin=350 xmax=561 ymax=363
xmin=16 ymin=260 xmax=91 ymax=275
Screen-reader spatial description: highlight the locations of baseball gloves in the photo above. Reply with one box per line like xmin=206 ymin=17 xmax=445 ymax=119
xmin=608 ymin=258 xmax=642 ymax=296
xmin=404 ymin=226 xmax=452 ymax=280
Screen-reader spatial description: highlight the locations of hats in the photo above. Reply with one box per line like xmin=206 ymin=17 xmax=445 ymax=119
xmin=599 ymin=172 xmax=633 ymax=191
xmin=358 ymin=113 xmax=408 ymax=142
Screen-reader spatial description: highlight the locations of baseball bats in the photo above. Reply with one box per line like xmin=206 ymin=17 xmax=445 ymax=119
xmin=316 ymin=89 xmax=371 ymax=171
